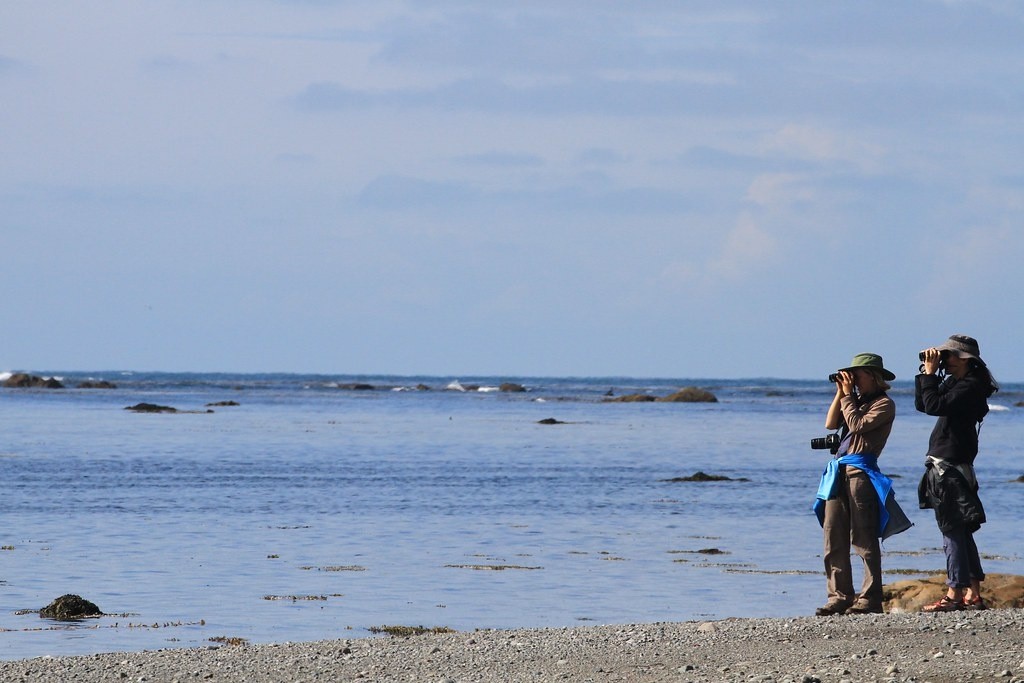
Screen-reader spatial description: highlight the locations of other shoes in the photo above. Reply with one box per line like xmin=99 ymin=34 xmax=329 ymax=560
xmin=816 ymin=603 xmax=850 ymax=616
xmin=845 ymin=597 xmax=883 ymax=615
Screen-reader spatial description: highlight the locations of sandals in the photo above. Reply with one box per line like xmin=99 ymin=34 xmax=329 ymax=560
xmin=922 ymin=595 xmax=964 ymax=612
xmin=962 ymin=595 xmax=986 ymax=610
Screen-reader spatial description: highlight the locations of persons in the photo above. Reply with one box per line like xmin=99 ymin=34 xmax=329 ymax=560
xmin=914 ymin=334 xmax=995 ymax=613
xmin=809 ymin=353 xmax=913 ymax=615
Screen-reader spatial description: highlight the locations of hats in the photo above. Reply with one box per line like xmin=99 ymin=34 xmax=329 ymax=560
xmin=838 ymin=353 xmax=896 ymax=381
xmin=935 ymin=335 xmax=986 ymax=366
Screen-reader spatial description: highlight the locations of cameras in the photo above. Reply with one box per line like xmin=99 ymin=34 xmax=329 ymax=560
xmin=828 ymin=373 xmax=843 ymax=382
xmin=919 ymin=350 xmax=949 ymax=361
xmin=811 ymin=433 xmax=841 ymax=454
